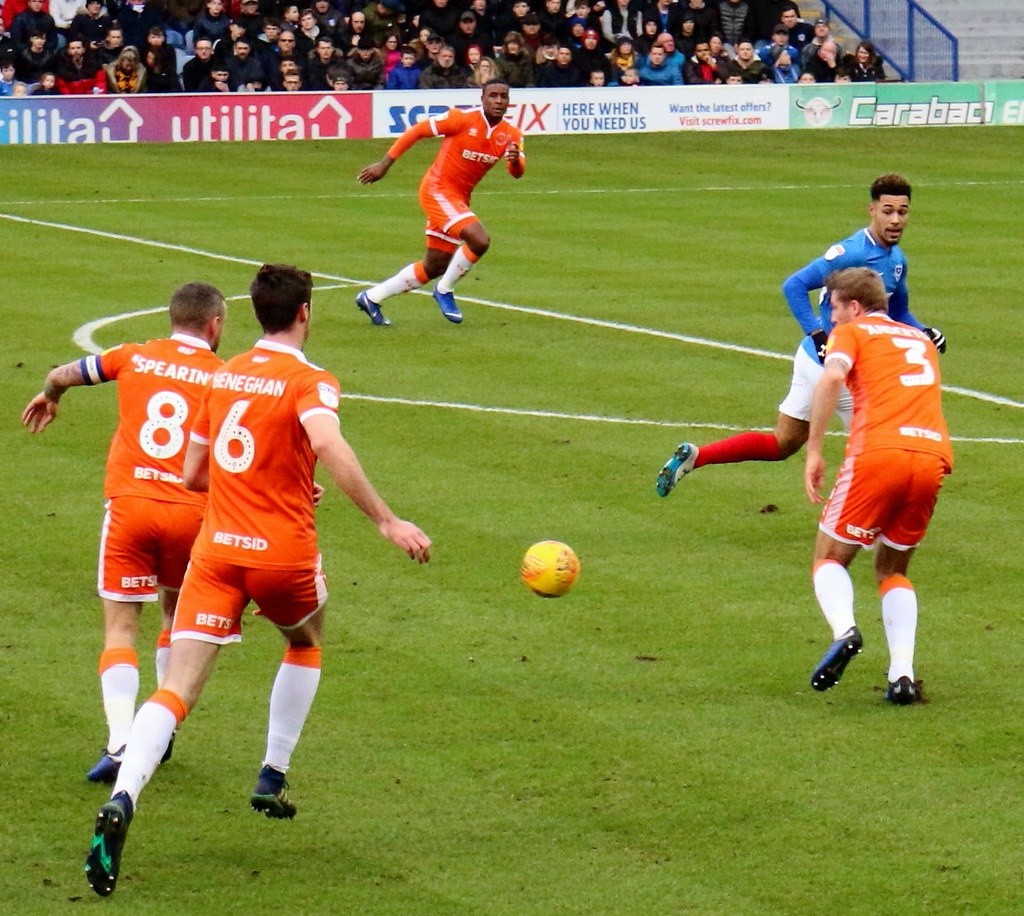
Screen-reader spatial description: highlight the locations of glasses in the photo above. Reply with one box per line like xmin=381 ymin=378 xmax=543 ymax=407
xmin=280 ymin=39 xmax=294 ymax=43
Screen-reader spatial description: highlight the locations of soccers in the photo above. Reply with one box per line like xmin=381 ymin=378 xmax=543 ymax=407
xmin=521 ymin=540 xmax=581 ymax=599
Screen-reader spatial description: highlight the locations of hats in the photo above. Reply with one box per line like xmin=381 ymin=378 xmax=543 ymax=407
xmin=381 ymin=0 xmax=406 ymax=12
xmin=358 ymin=38 xmax=373 ymax=49
xmin=815 ymin=18 xmax=827 ymax=26
xmin=461 ymin=12 xmax=476 ymax=20
xmin=570 ymin=18 xmax=587 ymax=28
xmin=772 ymin=47 xmax=786 ymax=60
xmin=504 ymin=31 xmax=524 ymax=47
xmin=581 ymin=32 xmax=600 ymax=44
xmin=773 ymin=24 xmax=788 ymax=33
xmin=522 ymin=14 xmax=539 ymax=24
xmin=334 ymin=70 xmax=352 ymax=86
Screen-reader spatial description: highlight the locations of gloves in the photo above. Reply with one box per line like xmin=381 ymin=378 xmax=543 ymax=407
xmin=924 ymin=327 xmax=952 ymax=354
xmin=813 ymin=330 xmax=828 ymax=364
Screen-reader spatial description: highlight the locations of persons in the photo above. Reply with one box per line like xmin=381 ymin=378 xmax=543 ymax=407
xmin=658 ymin=174 xmax=947 ymax=497
xmin=355 ymin=79 xmax=526 ymax=327
xmin=804 ymin=266 xmax=953 ymax=703
xmin=24 ymin=283 xmax=229 ymax=783
xmin=86 ymin=262 xmax=430 ymax=896
xmin=0 ymin=0 xmax=887 ymax=95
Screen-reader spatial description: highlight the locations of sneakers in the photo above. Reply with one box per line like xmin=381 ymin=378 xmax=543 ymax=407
xmin=160 ymin=734 xmax=176 ymax=763
xmin=251 ymin=766 xmax=296 ymax=820
xmin=433 ymin=286 xmax=463 ymax=324
xmin=811 ymin=626 xmax=865 ymax=692
xmin=656 ymin=442 xmax=699 ymax=497
xmin=88 ymin=745 xmax=130 ymax=781
xmin=87 ymin=792 xmax=138 ymax=897
xmin=355 ymin=291 xmax=391 ymax=326
xmin=886 ymin=678 xmax=917 ymax=702
